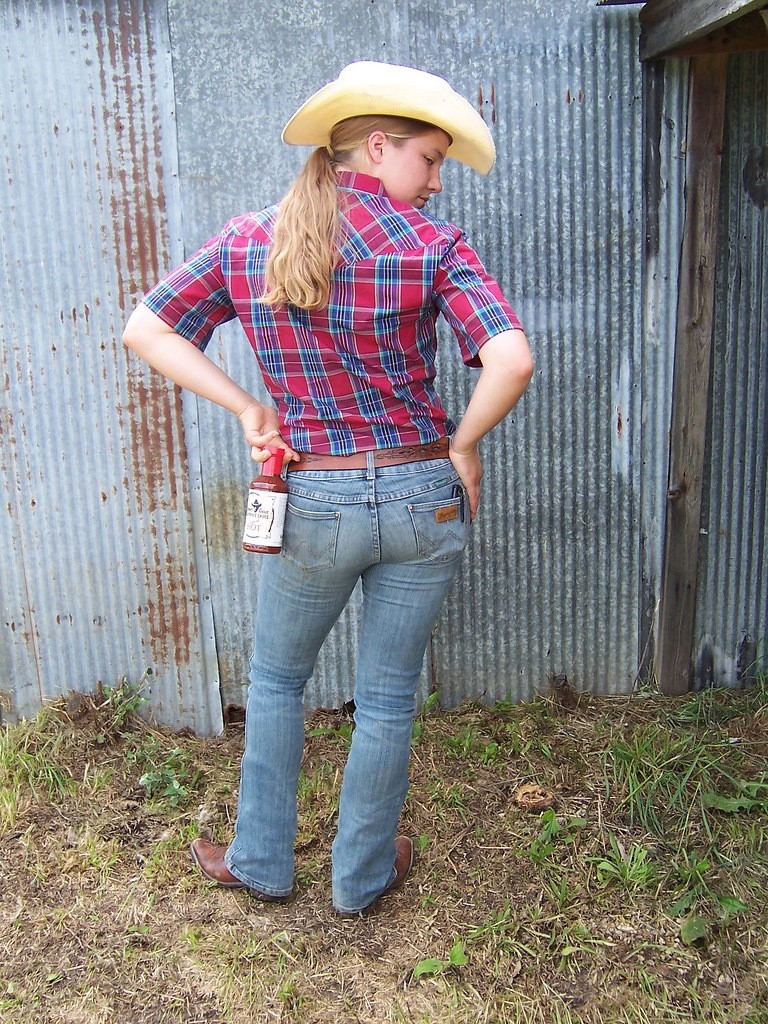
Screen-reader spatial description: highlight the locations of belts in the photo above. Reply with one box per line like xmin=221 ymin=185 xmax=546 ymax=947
xmin=286 ymin=436 xmax=452 ymax=472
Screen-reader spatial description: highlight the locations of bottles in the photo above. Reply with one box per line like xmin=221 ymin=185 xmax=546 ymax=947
xmin=241 ymin=446 xmax=289 ymax=554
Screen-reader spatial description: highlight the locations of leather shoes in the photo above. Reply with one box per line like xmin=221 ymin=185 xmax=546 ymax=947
xmin=351 ymin=836 xmax=414 ymax=916
xmin=190 ymin=838 xmax=278 ymax=902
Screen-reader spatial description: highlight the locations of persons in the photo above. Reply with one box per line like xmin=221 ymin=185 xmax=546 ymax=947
xmin=120 ymin=61 xmax=534 ymax=915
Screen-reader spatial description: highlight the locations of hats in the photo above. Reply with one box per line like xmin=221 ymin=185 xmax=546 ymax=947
xmin=281 ymin=61 xmax=496 ymax=174
